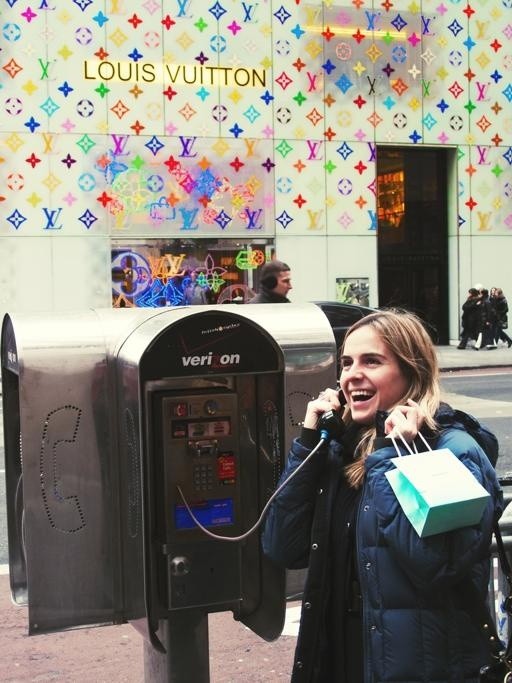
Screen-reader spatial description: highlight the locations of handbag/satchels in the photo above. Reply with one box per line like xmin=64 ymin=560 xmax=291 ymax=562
xmin=384 ymin=425 xmax=490 ymax=539
xmin=478 ymin=594 xmax=512 ymax=682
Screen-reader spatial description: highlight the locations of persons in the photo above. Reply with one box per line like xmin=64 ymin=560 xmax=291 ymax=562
xmin=260 ymin=306 xmax=504 ymax=683
xmin=248 ymin=261 xmax=292 ymax=303
xmin=456 ymin=288 xmax=511 ymax=350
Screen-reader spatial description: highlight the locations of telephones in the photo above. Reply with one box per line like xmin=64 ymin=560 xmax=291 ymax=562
xmin=316 ymin=386 xmax=347 ymax=439
